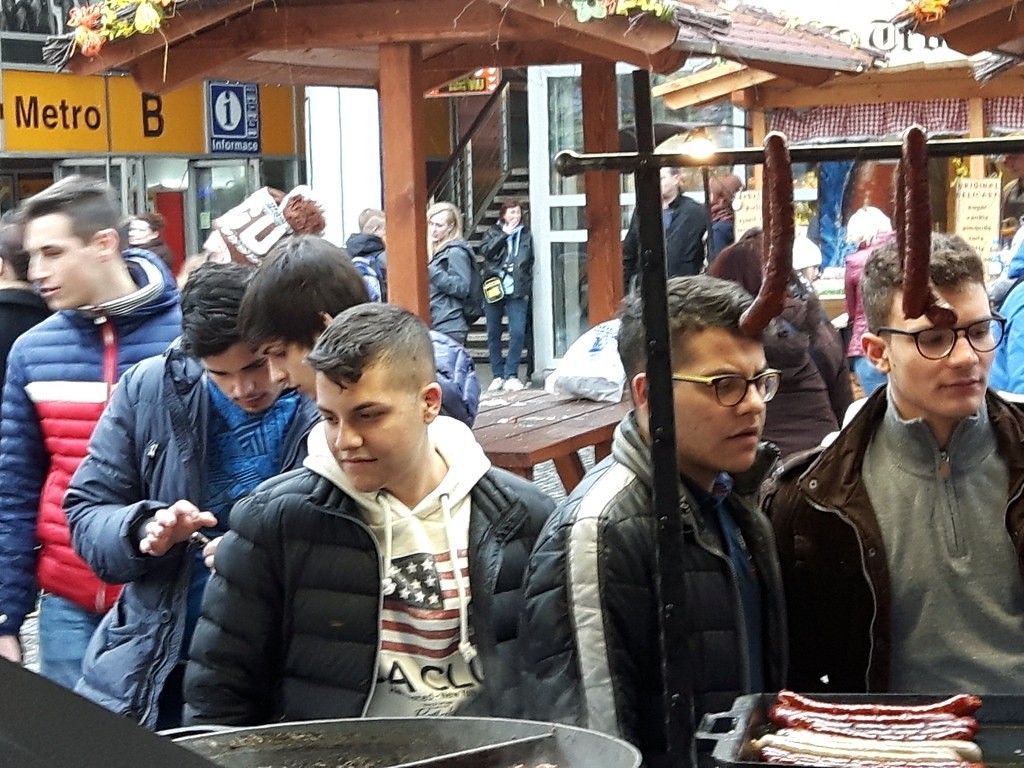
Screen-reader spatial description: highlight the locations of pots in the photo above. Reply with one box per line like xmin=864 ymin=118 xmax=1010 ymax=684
xmin=155 ymin=714 xmax=641 ymax=768
xmin=695 ymin=694 xmax=1024 ymax=768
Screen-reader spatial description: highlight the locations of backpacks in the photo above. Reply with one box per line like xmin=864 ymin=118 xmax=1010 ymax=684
xmin=434 ymin=239 xmax=485 ymax=324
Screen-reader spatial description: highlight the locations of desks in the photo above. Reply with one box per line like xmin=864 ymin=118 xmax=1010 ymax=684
xmin=470 ymin=387 xmax=635 ymax=495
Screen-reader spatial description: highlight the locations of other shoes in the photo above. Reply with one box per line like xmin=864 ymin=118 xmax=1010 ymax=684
xmin=503 ymin=376 xmax=523 ymax=391
xmin=488 ymin=377 xmax=505 ymax=392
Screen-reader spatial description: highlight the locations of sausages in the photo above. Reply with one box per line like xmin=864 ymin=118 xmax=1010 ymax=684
xmin=751 ymin=689 xmax=988 ymax=768
xmin=738 ymin=131 xmax=794 ymax=334
xmin=892 ymin=126 xmax=959 ymax=329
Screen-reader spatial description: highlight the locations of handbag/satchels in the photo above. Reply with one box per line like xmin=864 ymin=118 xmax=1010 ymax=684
xmin=482 ymin=276 xmax=505 ymax=304
xmin=544 ymin=318 xmax=628 ymax=402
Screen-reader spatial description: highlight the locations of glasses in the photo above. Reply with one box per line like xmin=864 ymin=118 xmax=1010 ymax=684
xmin=672 ymin=368 xmax=786 ymax=407
xmin=876 ymin=310 xmax=1007 ymax=360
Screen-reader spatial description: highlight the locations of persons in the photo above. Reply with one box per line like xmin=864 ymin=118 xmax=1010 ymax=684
xmin=480 ymin=200 xmax=535 ymax=392
xmin=341 ymin=208 xmax=387 ymax=303
xmin=184 ymin=303 xmax=556 ymax=725
xmin=425 ymin=201 xmax=475 ymax=344
xmin=708 ymin=173 xmax=740 ymax=275
xmin=713 ymin=226 xmax=854 ymax=461
xmin=845 ymin=207 xmax=897 ymax=394
xmin=0 ymin=174 xmax=182 ymax=690
xmin=0 ymin=211 xmax=54 ymax=388
xmin=985 ymin=230 xmax=1024 ymax=398
xmin=622 ymin=167 xmax=706 ymax=294
xmin=128 ymin=212 xmax=172 ymax=269
xmin=66 ymin=233 xmax=324 ymax=735
xmin=766 ymin=231 xmax=1024 ymax=696
xmin=235 ymin=232 xmax=480 ymax=429
xmin=518 ymin=274 xmax=787 ymax=768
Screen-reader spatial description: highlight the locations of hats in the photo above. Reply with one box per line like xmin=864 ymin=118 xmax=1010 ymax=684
xmin=847 ymin=205 xmax=892 ymax=244
xmin=793 ymin=238 xmax=822 ymax=270
